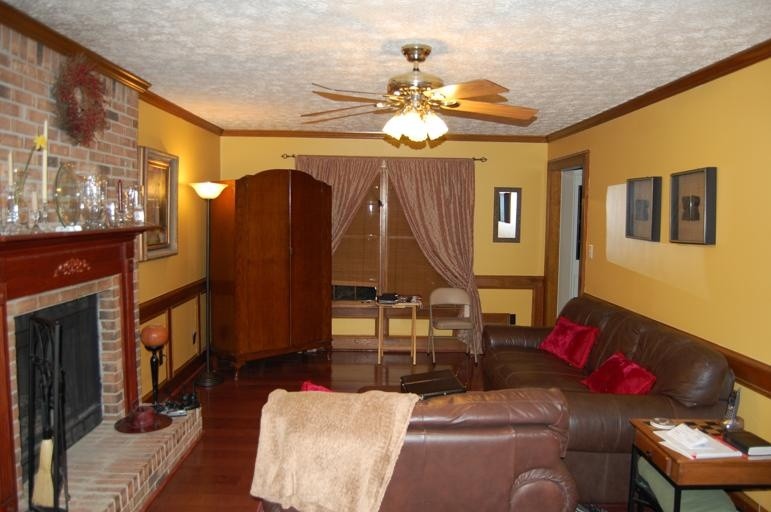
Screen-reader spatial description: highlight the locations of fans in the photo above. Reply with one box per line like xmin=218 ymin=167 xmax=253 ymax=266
xmin=299 ymin=43 xmax=538 ymax=124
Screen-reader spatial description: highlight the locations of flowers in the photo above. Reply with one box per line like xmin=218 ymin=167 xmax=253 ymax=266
xmin=19 ymin=135 xmax=48 ymax=170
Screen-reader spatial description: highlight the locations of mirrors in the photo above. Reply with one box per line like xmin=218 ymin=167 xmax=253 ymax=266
xmin=491 ymin=186 xmax=522 ymax=243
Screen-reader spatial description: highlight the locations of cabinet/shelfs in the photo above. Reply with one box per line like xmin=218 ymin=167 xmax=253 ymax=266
xmin=208 ymin=168 xmax=332 ymax=381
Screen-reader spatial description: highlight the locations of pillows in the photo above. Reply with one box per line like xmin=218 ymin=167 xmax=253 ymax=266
xmin=537 ymin=317 xmax=598 ymax=366
xmin=578 ymin=352 xmax=657 ymax=396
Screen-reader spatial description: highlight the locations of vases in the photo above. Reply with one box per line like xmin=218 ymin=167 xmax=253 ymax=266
xmin=0 ymin=167 xmax=39 ymax=233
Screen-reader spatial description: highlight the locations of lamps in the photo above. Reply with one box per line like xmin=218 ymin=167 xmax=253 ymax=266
xmin=140 ymin=324 xmax=168 ymax=413
xmin=189 ymin=180 xmax=228 ymax=389
xmin=378 ymin=105 xmax=449 ymax=146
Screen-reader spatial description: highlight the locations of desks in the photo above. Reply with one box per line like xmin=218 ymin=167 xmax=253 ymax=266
xmin=375 ymin=299 xmax=417 ymax=366
xmin=628 ymin=414 xmax=771 ymax=512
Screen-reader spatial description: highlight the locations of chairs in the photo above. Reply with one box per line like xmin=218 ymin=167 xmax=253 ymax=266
xmin=425 ymin=287 xmax=479 ymax=366
xmin=27 ymin=312 xmax=66 ymax=511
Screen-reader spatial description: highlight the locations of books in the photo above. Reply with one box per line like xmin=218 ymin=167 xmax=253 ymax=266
xmin=379 ymin=293 xmax=397 ymax=304
xmin=653 ymin=426 xmax=743 ymax=460
xmin=723 ymin=431 xmax=770 ymax=457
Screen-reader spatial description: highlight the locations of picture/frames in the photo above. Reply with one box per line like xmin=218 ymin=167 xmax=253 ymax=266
xmin=136 ymin=142 xmax=179 ymax=262
xmin=623 ymin=176 xmax=660 ymax=242
xmin=670 ymin=167 xmax=716 ymax=244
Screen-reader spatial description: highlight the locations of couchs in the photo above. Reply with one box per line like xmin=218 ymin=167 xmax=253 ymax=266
xmin=479 ymin=294 xmax=734 ymax=504
xmin=261 ymin=390 xmax=576 ymax=512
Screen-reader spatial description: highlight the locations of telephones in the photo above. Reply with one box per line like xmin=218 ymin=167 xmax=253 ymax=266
xmin=721 ymin=388 xmax=744 ymax=433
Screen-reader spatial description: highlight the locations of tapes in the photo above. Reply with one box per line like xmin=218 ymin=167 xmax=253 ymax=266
xmin=651 ymin=416 xmax=676 ymax=430
